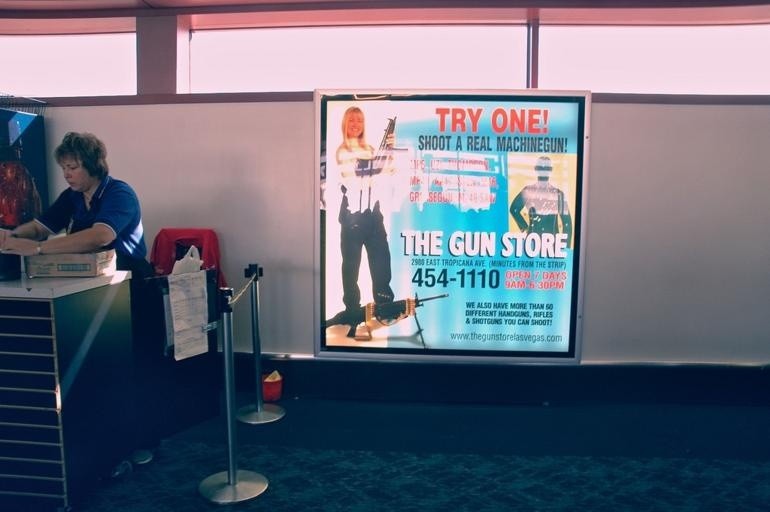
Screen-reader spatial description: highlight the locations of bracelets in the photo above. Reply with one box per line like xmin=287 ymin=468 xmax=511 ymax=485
xmin=36 ymin=241 xmax=42 ymax=255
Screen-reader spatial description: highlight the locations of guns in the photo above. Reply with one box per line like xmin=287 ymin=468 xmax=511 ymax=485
xmin=373 ymin=117 xmax=409 ymax=169
xmin=325 ymin=292 xmax=449 ymax=348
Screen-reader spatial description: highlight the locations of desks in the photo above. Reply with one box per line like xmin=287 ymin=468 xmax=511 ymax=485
xmin=1 ymin=270 xmax=137 ymax=495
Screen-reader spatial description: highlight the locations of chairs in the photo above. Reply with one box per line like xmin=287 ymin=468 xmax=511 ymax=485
xmin=148 ymin=228 xmax=218 ymax=279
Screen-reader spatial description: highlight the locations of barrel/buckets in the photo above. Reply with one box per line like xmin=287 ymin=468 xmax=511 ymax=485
xmin=262 ymin=374 xmax=284 ymax=403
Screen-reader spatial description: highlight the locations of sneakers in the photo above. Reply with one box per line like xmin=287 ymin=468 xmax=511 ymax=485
xmin=109 ymin=449 xmax=153 ymax=479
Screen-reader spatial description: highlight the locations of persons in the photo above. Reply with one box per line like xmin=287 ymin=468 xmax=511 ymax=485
xmin=335 ymin=106 xmax=394 ymax=338
xmin=0 ymin=132 xmax=160 ymax=465
xmin=510 ymin=156 xmax=573 ymax=249
